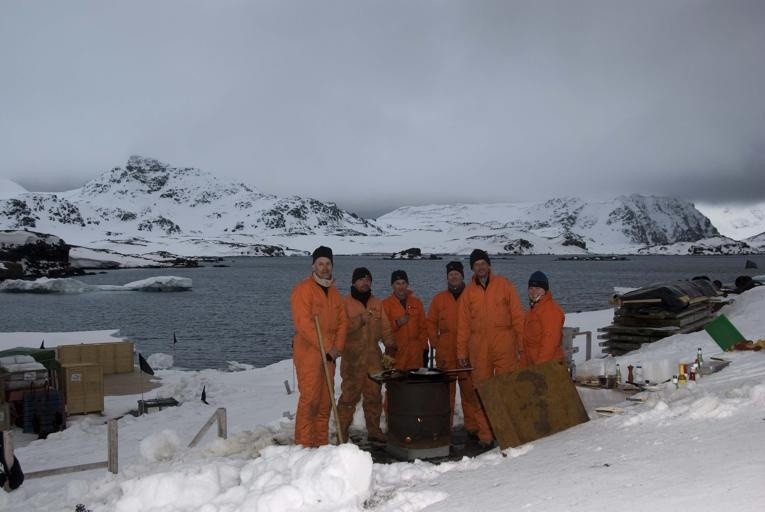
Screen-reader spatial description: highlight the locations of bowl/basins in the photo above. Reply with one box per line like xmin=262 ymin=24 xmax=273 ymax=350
xmin=598 ymin=375 xmax=615 ymax=388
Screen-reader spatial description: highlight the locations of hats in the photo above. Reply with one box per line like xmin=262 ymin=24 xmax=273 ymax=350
xmin=529 ymin=271 xmax=548 ymax=291
xmin=470 ymin=249 xmax=491 ymax=269
xmin=352 ymin=268 xmax=372 ymax=283
xmin=313 ymin=246 xmax=332 ymax=264
xmin=447 ymin=261 xmax=464 ymax=275
xmin=391 ymin=271 xmax=408 ymax=285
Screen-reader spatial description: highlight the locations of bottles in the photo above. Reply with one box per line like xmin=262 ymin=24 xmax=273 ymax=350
xmin=670 ymin=348 xmax=704 ymax=389
xmin=615 ymin=363 xmax=649 ymax=388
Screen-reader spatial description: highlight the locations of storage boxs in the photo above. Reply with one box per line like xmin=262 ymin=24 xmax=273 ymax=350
xmin=57 ymin=342 xmax=135 ymax=415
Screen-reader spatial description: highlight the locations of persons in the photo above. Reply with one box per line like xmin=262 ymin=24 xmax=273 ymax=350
xmin=334 ymin=266 xmax=400 ymax=446
xmin=455 ymin=247 xmax=526 ymax=448
xmin=292 ymin=245 xmax=348 ymax=448
xmin=520 ymin=267 xmax=565 ymax=371
xmin=425 ymin=259 xmax=478 ymax=434
xmin=379 ymin=268 xmax=428 ymax=419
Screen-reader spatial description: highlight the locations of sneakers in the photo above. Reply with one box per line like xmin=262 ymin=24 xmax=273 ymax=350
xmin=368 ymin=428 xmax=386 ymax=442
